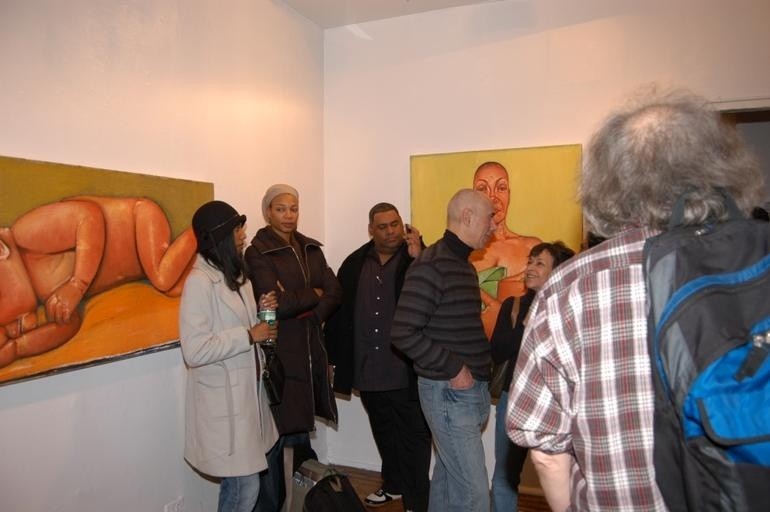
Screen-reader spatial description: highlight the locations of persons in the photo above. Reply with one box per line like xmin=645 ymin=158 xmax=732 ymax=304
xmin=443 ymin=159 xmax=550 ymax=344
xmin=486 ymin=239 xmax=575 ymax=511
xmin=177 ymin=199 xmax=287 ymax=512
xmin=501 ymin=88 xmax=769 ymax=510
xmin=324 ymin=202 xmax=433 ymax=510
xmin=241 ymin=184 xmax=342 ymax=471
xmin=387 ymin=187 xmax=496 ymax=512
xmin=1 ymin=191 xmax=205 ymax=369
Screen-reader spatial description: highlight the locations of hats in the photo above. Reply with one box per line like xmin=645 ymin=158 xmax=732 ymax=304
xmin=261 ymin=183 xmax=301 ymax=224
xmin=191 ymin=201 xmax=248 ymax=239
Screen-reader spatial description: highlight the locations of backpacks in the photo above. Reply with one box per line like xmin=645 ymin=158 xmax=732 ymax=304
xmin=284 ymin=457 xmax=335 ymax=512
xmin=301 ymin=473 xmax=368 ymax=512
xmin=640 ymin=182 xmax=770 ymax=512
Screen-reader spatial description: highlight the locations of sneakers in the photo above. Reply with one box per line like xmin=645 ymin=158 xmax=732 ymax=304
xmin=364 ymin=489 xmax=402 ymax=507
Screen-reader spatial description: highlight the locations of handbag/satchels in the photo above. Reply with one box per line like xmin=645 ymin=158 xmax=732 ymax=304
xmin=488 ymin=359 xmax=512 ymax=400
xmin=264 ymin=353 xmax=289 ymax=406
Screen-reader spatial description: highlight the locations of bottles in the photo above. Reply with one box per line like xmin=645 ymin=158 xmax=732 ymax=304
xmin=258 ymin=296 xmax=278 ymax=347
